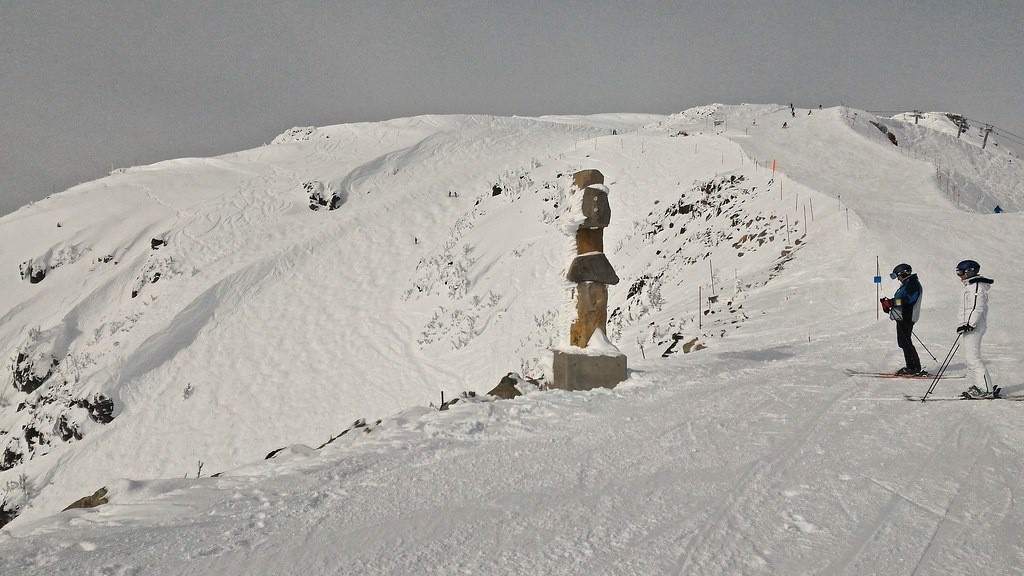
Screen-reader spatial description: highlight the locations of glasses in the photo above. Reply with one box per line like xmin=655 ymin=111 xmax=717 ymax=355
xmin=890 ymin=273 xmax=897 ymax=279
xmin=956 ymin=269 xmax=966 ymax=277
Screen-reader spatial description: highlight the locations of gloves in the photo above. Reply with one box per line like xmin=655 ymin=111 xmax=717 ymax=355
xmin=888 ymin=298 xmax=895 ymax=307
xmin=957 ymin=325 xmax=974 ymax=335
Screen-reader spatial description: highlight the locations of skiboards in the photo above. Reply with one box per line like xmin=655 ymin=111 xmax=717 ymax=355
xmin=901 ymin=391 xmax=1024 ymax=402
xmin=842 ymin=367 xmax=967 ymax=379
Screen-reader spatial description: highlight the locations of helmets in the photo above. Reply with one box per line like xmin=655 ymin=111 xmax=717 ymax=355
xmin=893 ymin=264 xmax=912 ymax=277
xmin=956 ymin=259 xmax=980 ymax=274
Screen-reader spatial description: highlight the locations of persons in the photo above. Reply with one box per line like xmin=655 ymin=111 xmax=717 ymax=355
xmin=956 ymin=260 xmax=994 ymax=400
xmin=879 ymin=263 xmax=924 ymax=377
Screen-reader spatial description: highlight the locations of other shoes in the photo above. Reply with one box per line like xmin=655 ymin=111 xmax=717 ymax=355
xmin=895 ymin=367 xmax=920 ymax=377
xmin=962 ymin=386 xmax=994 ymax=399
